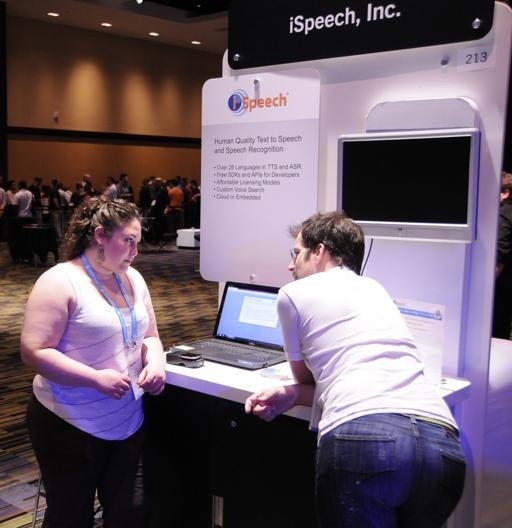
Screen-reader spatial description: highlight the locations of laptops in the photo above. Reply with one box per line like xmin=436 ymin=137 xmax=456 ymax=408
xmin=183 ymin=281 xmax=287 ymax=370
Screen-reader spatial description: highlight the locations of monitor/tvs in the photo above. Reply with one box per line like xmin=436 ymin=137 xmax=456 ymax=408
xmin=336 ymin=130 xmax=480 ymax=239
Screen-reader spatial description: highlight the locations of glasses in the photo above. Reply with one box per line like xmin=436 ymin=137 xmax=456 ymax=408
xmin=287 ymin=247 xmax=300 ymax=262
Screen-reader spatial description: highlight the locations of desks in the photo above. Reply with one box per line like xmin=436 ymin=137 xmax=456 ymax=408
xmin=146 ymin=338 xmax=473 ymax=528
xmin=18 ymin=223 xmax=59 ymax=270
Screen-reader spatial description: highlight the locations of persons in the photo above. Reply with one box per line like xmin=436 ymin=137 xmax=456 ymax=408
xmin=190 ymin=186 xmax=200 ymax=215
xmin=244 ymin=211 xmax=468 ymax=526
xmin=6 ymin=179 xmax=17 ymax=217
xmin=116 ymin=173 xmax=133 ymax=204
xmin=20 ymin=195 xmax=168 ymax=528
xmin=182 ymin=176 xmax=192 ymax=193
xmin=0 ymin=186 xmax=7 ymax=241
xmin=147 ymin=176 xmax=170 ymax=242
xmin=139 ymin=177 xmax=152 ymax=209
xmin=70 ymin=183 xmax=86 ymax=206
xmin=102 ymin=176 xmax=117 ymax=198
xmin=175 ymin=176 xmax=183 ymax=188
xmin=81 ymin=174 xmax=91 ymax=190
xmin=164 ymin=179 xmax=186 ymax=228
xmin=490 ymin=169 xmax=512 ymax=340
xmin=27 ymin=177 xmax=51 ymax=224
xmin=48 ymin=178 xmax=61 ymax=238
xmin=57 ymin=182 xmax=74 ymax=209
xmin=12 ymin=180 xmax=35 ymax=217
